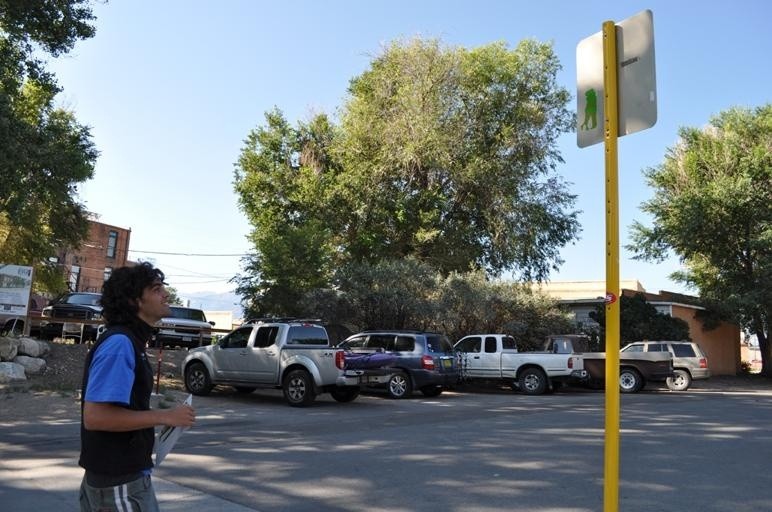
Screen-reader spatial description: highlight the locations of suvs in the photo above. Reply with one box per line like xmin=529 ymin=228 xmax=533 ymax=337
xmin=619 ymin=340 xmax=707 ymax=391
xmin=329 ymin=328 xmax=463 ymax=401
xmin=0 ymin=283 xmax=216 ymax=352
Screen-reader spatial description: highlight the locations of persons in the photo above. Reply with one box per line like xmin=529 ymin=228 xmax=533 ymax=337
xmin=80 ymin=262 xmax=196 ymax=511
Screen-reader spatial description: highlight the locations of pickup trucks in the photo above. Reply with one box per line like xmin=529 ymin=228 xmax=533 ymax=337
xmin=182 ymin=321 xmax=345 ymax=400
xmin=452 ymin=333 xmax=584 ymax=394
xmin=543 ymin=334 xmax=673 ymax=394
